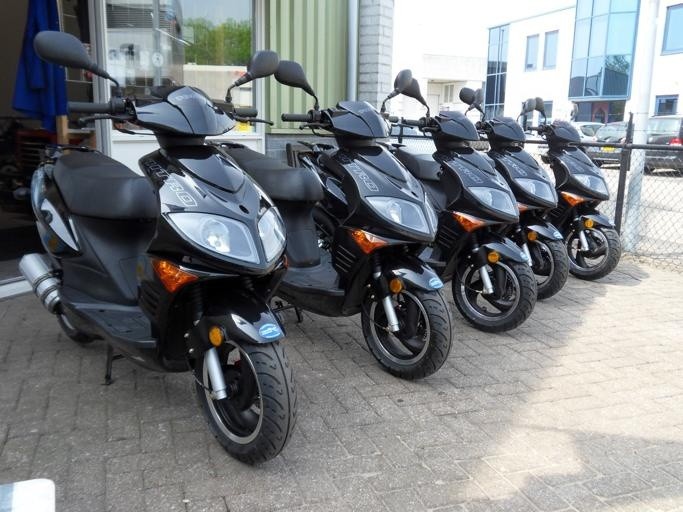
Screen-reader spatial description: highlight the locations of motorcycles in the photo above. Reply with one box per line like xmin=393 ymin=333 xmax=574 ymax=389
xmin=211 ymin=59 xmax=454 ymax=382
xmin=458 ymin=87 xmax=571 ymax=301
xmin=526 ymin=96 xmax=621 ymax=279
xmin=16 ymin=25 xmax=298 ymax=467
xmin=294 ymin=78 xmax=538 ymax=331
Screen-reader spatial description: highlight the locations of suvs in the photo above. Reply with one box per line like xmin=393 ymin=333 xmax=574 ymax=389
xmin=642 ymin=115 xmax=683 ymax=176
xmin=587 ymin=120 xmax=633 ymax=170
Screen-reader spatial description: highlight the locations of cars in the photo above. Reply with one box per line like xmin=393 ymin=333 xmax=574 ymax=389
xmin=537 ymin=122 xmax=605 ymax=163
